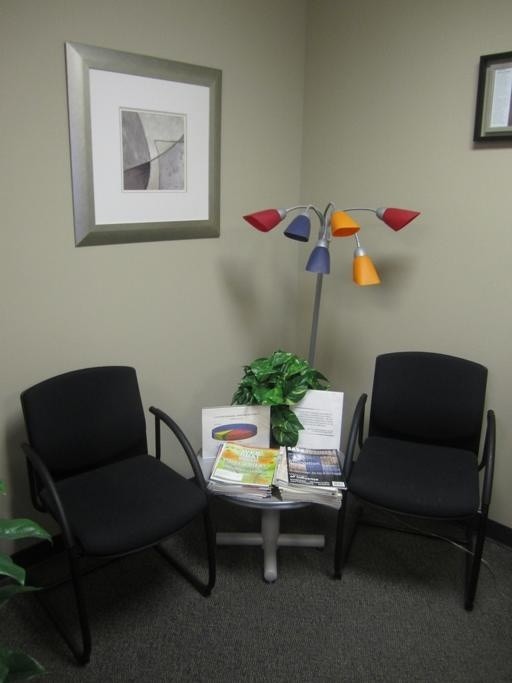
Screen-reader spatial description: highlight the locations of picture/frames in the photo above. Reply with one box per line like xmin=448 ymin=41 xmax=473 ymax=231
xmin=473 ymin=51 xmax=512 ymax=143
xmin=63 ymin=40 xmax=223 ymax=247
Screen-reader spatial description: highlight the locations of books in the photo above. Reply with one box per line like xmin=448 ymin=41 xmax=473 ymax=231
xmin=207 ymin=442 xmax=279 ymax=501
xmin=273 ymin=445 xmax=348 ymax=509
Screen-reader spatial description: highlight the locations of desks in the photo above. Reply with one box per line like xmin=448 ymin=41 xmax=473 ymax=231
xmin=197 ymin=448 xmax=326 ymax=581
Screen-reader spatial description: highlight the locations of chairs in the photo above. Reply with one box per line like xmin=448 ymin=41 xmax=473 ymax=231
xmin=334 ymin=351 xmax=496 ymax=611
xmin=19 ymin=364 xmax=218 ymax=665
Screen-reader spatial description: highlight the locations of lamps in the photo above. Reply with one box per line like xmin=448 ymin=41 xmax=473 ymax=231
xmin=243 ymin=203 xmax=421 ymax=367
